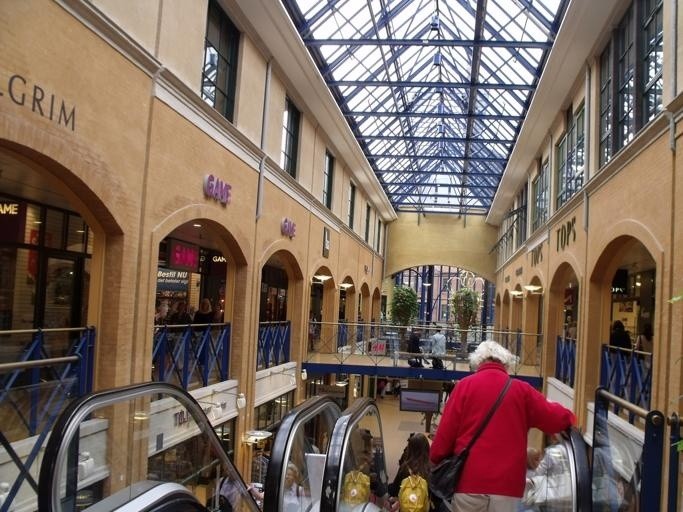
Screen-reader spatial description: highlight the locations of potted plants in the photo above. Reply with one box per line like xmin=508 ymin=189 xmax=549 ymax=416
xmin=389 ymin=284 xmax=480 ymax=359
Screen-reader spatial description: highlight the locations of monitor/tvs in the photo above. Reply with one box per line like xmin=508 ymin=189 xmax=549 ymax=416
xmin=400 ymin=388 xmax=440 ymax=413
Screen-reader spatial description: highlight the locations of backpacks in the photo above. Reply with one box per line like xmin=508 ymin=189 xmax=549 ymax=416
xmin=342 ymin=465 xmax=372 ymax=506
xmin=398 ymin=466 xmax=430 ymax=511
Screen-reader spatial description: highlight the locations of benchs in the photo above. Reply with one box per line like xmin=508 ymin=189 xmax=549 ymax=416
xmin=391 ymin=350 xmax=458 ymax=373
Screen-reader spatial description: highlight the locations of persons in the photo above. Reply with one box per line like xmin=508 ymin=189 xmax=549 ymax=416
xmin=634 ymin=322 xmax=653 ymax=373
xmin=245 ymin=460 xmax=308 ymax=512
xmin=440 ymin=381 xmax=456 ymax=404
xmin=168 ymin=301 xmax=193 ymax=373
xmin=151 ymin=304 xmax=164 ymax=368
xmin=408 ymin=326 xmax=428 ymax=367
xmin=193 ymin=298 xmax=214 ymax=368
xmin=515 ymin=446 xmax=544 ymax=512
xmin=336 ymin=428 xmax=390 ymax=512
xmin=431 ymin=326 xmax=446 ymax=354
xmin=524 ymin=433 xmax=576 ymax=512
xmin=207 ymin=460 xmax=242 ymax=512
xmin=609 ymin=320 xmax=630 ymax=373
xmin=208 ymin=301 xmax=223 ymax=361
xmin=394 ymin=379 xmax=401 ymax=398
xmin=387 ymin=432 xmax=431 ymax=512
xmin=428 ymin=338 xmax=576 ymax=512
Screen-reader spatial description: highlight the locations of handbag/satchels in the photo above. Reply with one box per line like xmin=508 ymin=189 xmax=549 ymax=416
xmin=546 ymin=450 xmax=573 ymax=502
xmin=427 ymin=454 xmax=467 ymax=505
xmin=592 ymin=451 xmax=616 ymax=505
